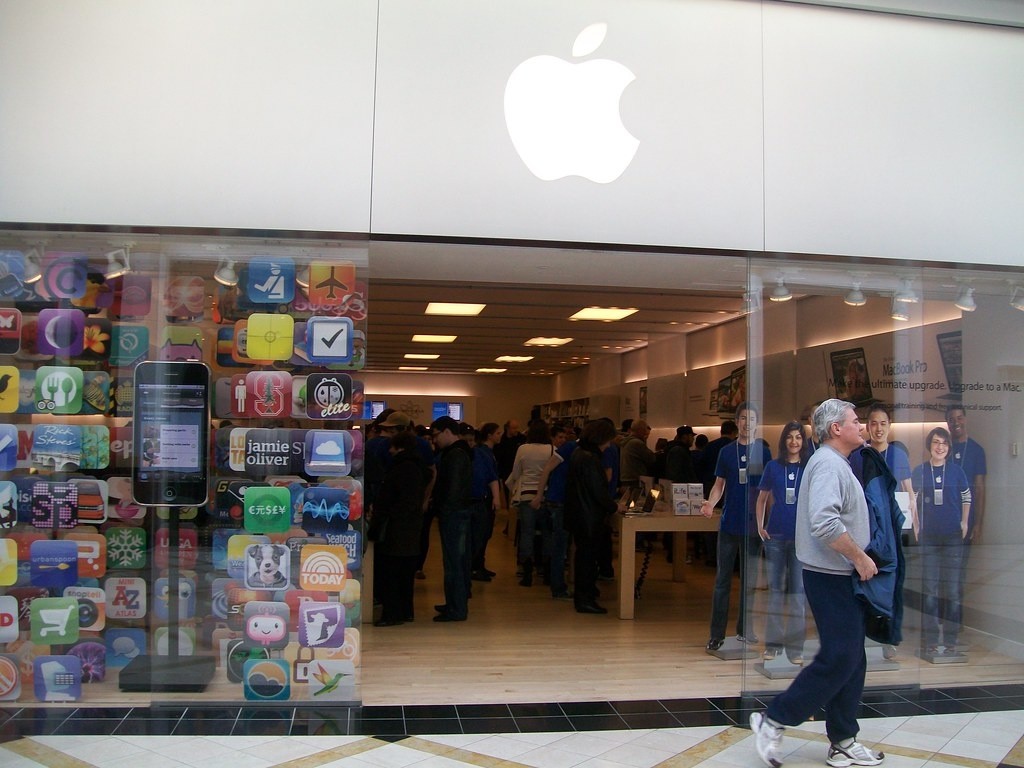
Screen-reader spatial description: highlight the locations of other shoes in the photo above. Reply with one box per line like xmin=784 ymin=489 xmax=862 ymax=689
xmin=516 ymin=566 xmax=523 ymax=576
xmin=374 ymin=613 xmax=414 ymax=627
xmin=922 ymin=643 xmax=956 ymax=656
xmin=471 ymin=568 xmax=496 ymax=581
xmin=553 ymin=592 xmax=574 ymax=602
xmin=519 ymin=577 xmax=532 ymax=586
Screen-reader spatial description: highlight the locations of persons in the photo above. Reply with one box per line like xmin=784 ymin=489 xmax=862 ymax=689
xmin=352 ymin=408 xmax=770 ymax=627
xmin=700 ymin=401 xmax=987 ymax=665
xmin=748 ymin=399 xmax=885 ymax=768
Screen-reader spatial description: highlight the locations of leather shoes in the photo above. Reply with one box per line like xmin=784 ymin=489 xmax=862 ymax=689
xmin=577 ymin=602 xmax=607 ymax=614
xmin=433 ymin=604 xmax=467 ymax=622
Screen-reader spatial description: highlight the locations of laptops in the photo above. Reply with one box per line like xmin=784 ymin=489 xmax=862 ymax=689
xmin=613 ymin=486 xmax=661 ymax=515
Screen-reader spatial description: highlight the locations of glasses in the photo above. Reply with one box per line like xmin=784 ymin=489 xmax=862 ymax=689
xmin=931 ymin=440 xmax=948 ymax=446
xmin=432 ymin=431 xmax=441 ymax=438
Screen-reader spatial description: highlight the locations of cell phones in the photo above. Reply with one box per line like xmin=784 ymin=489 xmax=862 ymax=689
xmin=131 ymin=359 xmax=212 ymax=507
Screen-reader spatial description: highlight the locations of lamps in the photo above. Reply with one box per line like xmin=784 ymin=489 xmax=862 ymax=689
xmin=214 ymin=255 xmax=240 ymax=286
xmin=768 ymin=276 xmax=793 ymax=302
xmin=22 ymin=238 xmax=47 ymax=283
xmin=896 ymin=278 xmax=919 ymax=304
xmin=954 ymin=284 xmax=977 ymax=312
xmin=296 ymin=265 xmax=310 ymax=287
xmin=105 ymin=241 xmax=135 ymax=279
xmin=891 ymin=299 xmax=911 ymax=322
xmin=1008 ymin=285 xmax=1024 ymax=311
xmin=843 ymin=279 xmax=867 ymax=306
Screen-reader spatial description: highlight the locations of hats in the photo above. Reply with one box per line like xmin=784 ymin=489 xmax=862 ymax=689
xmin=677 ymin=425 xmax=697 ymax=436
xmin=459 ymin=422 xmax=474 ymax=434
xmin=378 ymin=411 xmax=410 ymax=427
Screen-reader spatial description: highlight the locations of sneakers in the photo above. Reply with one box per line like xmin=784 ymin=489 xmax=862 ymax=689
xmin=749 ymin=712 xmax=786 ymax=768
xmin=736 ymin=634 xmax=759 ymax=644
xmin=825 ymin=740 xmax=885 ymax=768
xmin=789 ymin=656 xmax=802 ymax=664
xmin=763 ymin=649 xmax=782 ymax=659
xmin=668 ymin=554 xmax=693 ymax=566
xmin=882 ymin=644 xmax=897 ymax=659
xmin=706 ymin=638 xmax=724 ymax=650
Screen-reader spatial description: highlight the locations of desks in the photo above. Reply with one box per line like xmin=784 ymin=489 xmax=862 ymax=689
xmin=613 ymin=504 xmax=723 ymax=620
